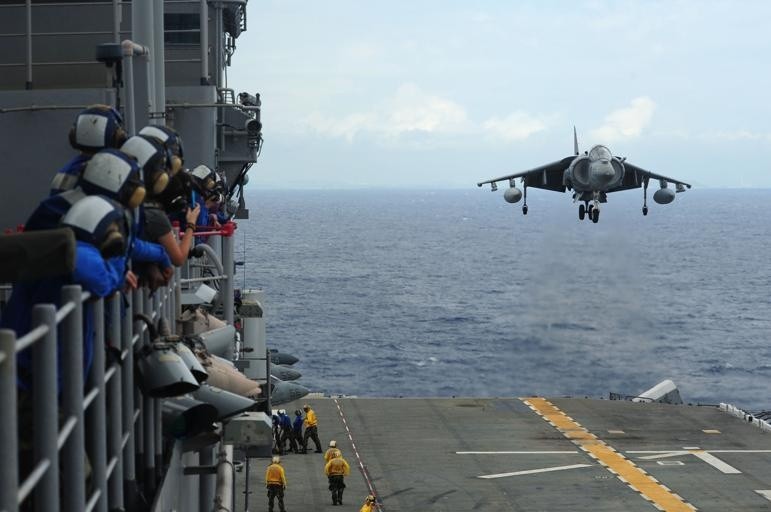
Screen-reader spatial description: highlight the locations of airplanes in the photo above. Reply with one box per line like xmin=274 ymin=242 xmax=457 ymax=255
xmin=477 ymin=126 xmax=691 ymax=224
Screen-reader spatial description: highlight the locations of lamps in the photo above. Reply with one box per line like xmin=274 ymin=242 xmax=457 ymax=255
xmin=245 ymin=118 xmax=262 ymax=135
xmin=131 ymin=304 xmax=262 ymax=440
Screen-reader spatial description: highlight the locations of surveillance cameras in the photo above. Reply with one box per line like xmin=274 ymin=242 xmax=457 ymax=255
xmin=245 ymin=119 xmax=262 ymax=134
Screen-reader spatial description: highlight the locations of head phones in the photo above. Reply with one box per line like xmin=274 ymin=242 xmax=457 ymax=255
xmin=67 ymin=103 xmax=223 ymax=261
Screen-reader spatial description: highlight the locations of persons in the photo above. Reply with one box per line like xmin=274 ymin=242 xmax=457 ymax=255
xmin=323 ymin=440 xmax=346 ymax=491
xmin=1 ymin=104 xmax=235 ymax=491
xmin=324 ymin=450 xmax=350 ymax=505
xmin=299 ymin=404 xmax=323 ymax=453
xmin=264 ymin=454 xmax=288 ymax=512
xmin=270 ymin=412 xmax=283 ymax=453
xmin=288 ymin=408 xmax=309 ymax=451
xmin=277 ymin=409 xmax=299 ymax=456
xmin=359 ymin=495 xmax=377 ymax=512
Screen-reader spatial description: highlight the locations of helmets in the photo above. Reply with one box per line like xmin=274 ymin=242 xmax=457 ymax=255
xmin=191 ymin=162 xmax=217 ymax=192
xmin=61 ymin=95 xmax=186 ymax=250
